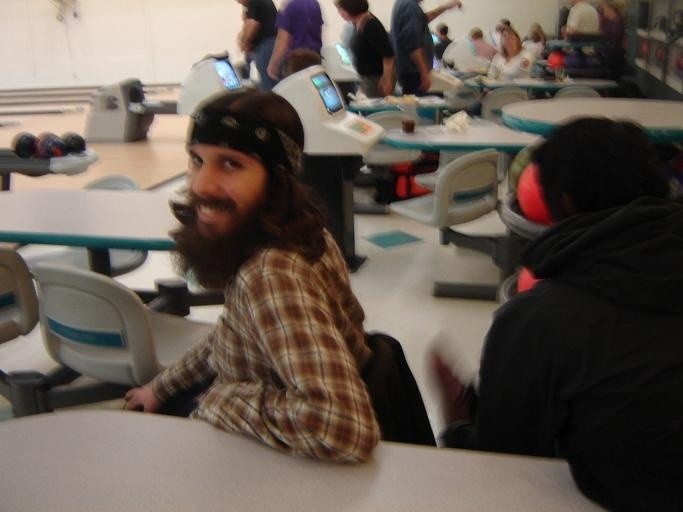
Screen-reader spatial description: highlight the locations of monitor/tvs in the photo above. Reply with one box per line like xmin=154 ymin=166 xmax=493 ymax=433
xmin=312 ymin=71 xmax=343 ymax=113
xmin=214 ymin=60 xmax=241 ymax=90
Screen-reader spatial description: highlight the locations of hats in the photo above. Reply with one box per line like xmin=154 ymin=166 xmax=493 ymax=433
xmin=567 ymin=2 xmax=600 ymax=33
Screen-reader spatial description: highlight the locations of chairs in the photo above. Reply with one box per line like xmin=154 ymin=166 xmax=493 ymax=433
xmin=0 ymin=41 xmax=683 ymax=316
xmin=0 ymin=250 xmax=40 ymax=345
xmin=35 ymin=261 xmax=219 ymax=388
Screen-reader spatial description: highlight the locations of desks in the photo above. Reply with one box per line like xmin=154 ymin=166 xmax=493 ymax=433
xmin=0 ymin=412 xmax=611 ymax=512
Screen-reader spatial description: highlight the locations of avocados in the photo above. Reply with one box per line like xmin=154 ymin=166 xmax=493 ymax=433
xmin=12 ymin=130 xmax=85 ymax=159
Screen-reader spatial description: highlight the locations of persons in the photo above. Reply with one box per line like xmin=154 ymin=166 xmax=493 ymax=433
xmin=267 ymin=0 xmax=323 ymax=81
xmin=425 ymin=118 xmax=682 ymax=512
xmin=434 ymin=1 xmax=627 ymax=97
xmin=389 ymin=0 xmax=463 ymax=98
xmin=234 ymin=1 xmax=279 ymax=89
xmin=124 ymin=87 xmax=398 ymax=465
xmin=336 ymin=1 xmax=395 ymax=103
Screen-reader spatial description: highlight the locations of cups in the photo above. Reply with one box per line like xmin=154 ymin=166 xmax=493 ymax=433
xmin=400 ymin=95 xmax=416 ymax=134
xmin=554 ymin=63 xmax=563 ymax=82
